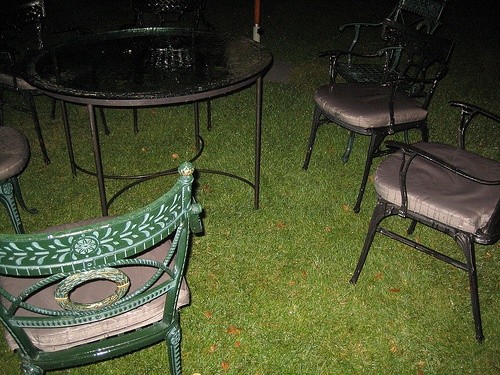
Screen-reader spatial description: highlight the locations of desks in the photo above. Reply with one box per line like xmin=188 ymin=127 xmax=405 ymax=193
xmin=21 ymin=27 xmax=274 ymax=219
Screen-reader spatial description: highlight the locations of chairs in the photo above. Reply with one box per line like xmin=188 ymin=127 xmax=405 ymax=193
xmin=0 ymin=162 xmax=203 ymax=375
xmin=133 ymin=0 xmax=212 ymax=150
xmin=303 ymin=0 xmax=500 ymax=344
xmin=0 ymin=125 xmax=38 ymax=235
xmin=0 ymin=0 xmax=111 ymax=164
